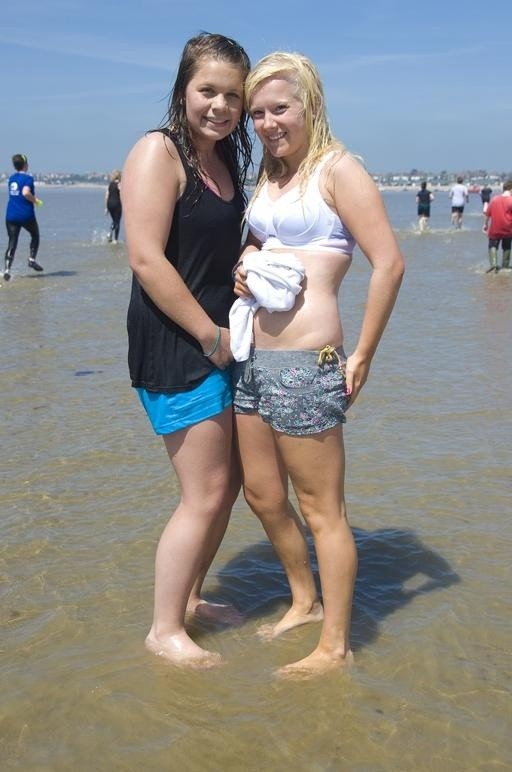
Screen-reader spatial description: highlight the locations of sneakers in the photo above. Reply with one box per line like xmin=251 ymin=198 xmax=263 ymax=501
xmin=4 ymin=273 xmax=10 ymax=279
xmin=29 ymin=261 xmax=42 ymax=271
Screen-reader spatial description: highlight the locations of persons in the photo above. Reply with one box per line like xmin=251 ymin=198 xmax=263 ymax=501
xmin=116 ymin=30 xmax=259 ymax=676
xmin=447 ymin=176 xmax=471 ymax=231
xmin=3 ymin=153 xmax=45 ymax=283
xmin=482 ymin=179 xmax=511 ymax=275
xmin=479 ymin=182 xmax=492 ymax=215
xmin=102 ymin=170 xmax=123 ymax=247
xmin=414 ymin=181 xmax=434 ymax=233
xmin=226 ymin=50 xmax=412 ymax=687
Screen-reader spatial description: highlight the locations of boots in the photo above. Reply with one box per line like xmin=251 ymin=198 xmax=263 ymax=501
xmin=486 ymin=246 xmax=497 ymax=272
xmin=503 ymin=249 xmax=512 ymax=269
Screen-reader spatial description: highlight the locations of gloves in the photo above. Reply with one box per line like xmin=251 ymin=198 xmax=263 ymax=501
xmin=35 ymin=200 xmax=42 ymax=208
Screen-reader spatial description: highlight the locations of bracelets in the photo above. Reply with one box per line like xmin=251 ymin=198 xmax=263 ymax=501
xmin=232 ymin=260 xmax=244 ymax=282
xmin=202 ymin=324 xmax=222 ymax=359
